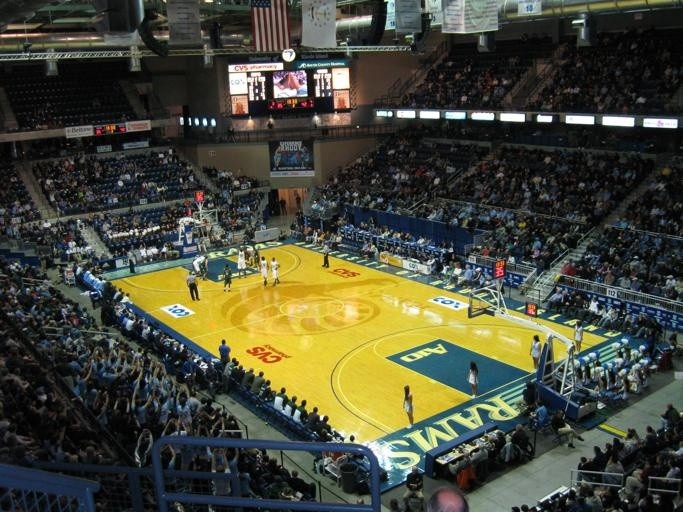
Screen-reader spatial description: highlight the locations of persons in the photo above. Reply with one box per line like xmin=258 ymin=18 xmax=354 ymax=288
xmin=3 ymin=20 xmax=679 ymax=510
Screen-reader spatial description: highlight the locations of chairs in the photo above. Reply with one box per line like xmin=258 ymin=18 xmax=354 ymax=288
xmin=424 ymin=420 xmax=528 ymax=492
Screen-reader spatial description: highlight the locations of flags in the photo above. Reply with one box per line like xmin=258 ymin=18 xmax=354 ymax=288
xmin=250 ymin=0 xmax=291 ymax=53
xmin=384 ymin=0 xmax=499 ymax=34
xmin=299 ymin=0 xmax=338 ymax=50
xmin=516 ymin=0 xmax=542 ymax=17
xmin=165 ymin=0 xmax=202 ymax=46
